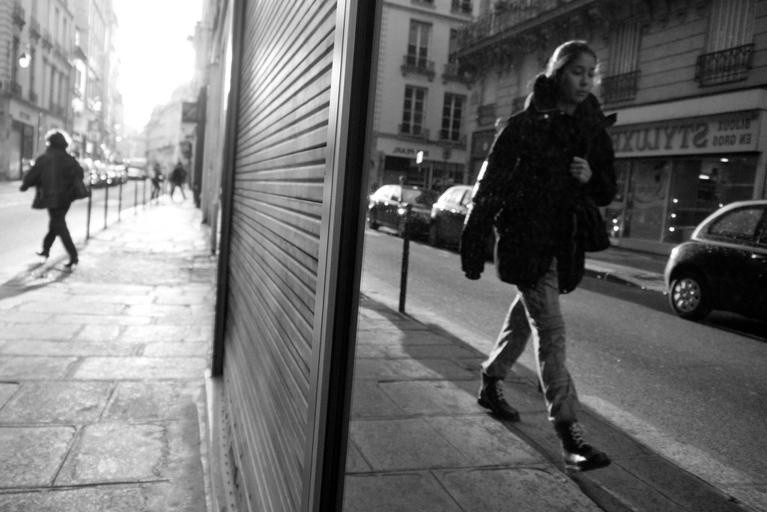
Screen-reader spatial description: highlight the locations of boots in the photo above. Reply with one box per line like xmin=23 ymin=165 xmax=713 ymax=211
xmin=554 ymin=417 xmax=611 ymax=472
xmin=478 ymin=372 xmax=519 ymax=422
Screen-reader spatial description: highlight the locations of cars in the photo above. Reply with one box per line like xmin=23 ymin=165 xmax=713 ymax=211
xmin=663 ymin=199 xmax=767 ymax=319
xmin=72 ymin=159 xmax=145 ymax=190
xmin=365 ymin=183 xmax=497 ymax=262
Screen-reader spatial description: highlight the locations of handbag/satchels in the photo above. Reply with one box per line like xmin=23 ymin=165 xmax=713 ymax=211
xmin=72 ymin=179 xmax=89 ymax=199
xmin=576 ymin=192 xmax=611 ymax=254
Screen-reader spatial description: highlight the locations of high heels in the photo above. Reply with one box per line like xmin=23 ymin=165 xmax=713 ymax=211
xmin=36 ymin=250 xmax=49 ymax=260
xmin=65 ymin=258 xmax=78 ymax=268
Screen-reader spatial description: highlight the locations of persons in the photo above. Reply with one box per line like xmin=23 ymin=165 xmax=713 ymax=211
xmin=168 ymin=160 xmax=189 ymax=199
xmin=456 ymin=39 xmax=619 ymax=475
xmin=150 ymin=162 xmax=165 ymax=200
xmin=19 ymin=128 xmax=90 ymax=268
